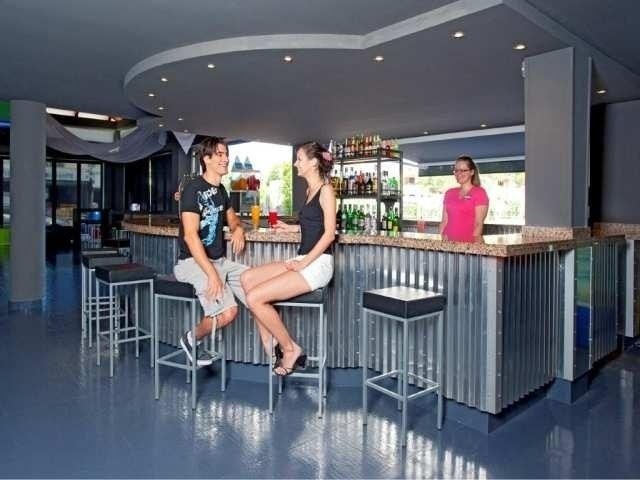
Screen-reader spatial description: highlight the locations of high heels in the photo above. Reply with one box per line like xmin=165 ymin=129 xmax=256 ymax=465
xmin=273 ymin=354 xmax=306 ymax=376
xmin=274 ymin=343 xmax=283 ymax=368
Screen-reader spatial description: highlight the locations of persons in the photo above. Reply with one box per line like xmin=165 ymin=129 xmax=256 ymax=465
xmin=172 ymin=137 xmax=281 ymax=368
xmin=239 ymin=141 xmax=336 ymax=377
xmin=438 ymin=154 xmax=489 ymax=237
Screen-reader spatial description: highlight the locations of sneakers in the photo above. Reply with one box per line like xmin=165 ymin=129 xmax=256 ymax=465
xmin=179 ymin=331 xmax=213 ymax=366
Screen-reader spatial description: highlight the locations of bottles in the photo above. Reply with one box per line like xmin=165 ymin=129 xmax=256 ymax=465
xmin=347 ymin=206 xmax=352 ymax=230
xmin=365 ymin=204 xmax=371 ymax=232
xmin=382 ymin=212 xmax=388 ymax=231
xmin=371 ymin=205 xmax=377 ymax=232
xmin=341 ymin=205 xmax=348 ymax=230
xmin=382 ymin=171 xmax=400 ymax=193
xmin=359 ymin=205 xmax=364 ymax=231
xmin=268 ymin=212 xmax=277 ymax=226
xmin=251 ymin=205 xmax=262 ymax=231
xmin=336 ymin=205 xmax=341 ymax=229
xmin=334 ymin=167 xmax=377 ymax=193
xmin=329 ymin=136 xmax=401 ymax=159
xmin=387 ymin=208 xmax=393 ymax=231
xmin=394 ymin=207 xmax=400 ymax=233
xmin=352 ymin=204 xmax=358 ymax=229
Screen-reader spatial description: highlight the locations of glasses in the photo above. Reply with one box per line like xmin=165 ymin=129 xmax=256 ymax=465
xmin=453 ymin=169 xmax=472 ymax=174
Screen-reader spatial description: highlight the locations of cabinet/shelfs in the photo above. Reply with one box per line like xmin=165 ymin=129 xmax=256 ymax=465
xmin=327 ymin=148 xmax=404 ymax=230
xmin=230 ymin=169 xmax=260 ymax=211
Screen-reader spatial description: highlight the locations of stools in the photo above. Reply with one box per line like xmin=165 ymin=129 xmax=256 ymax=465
xmin=268 ymin=287 xmax=329 ymax=419
xmin=81 ymin=246 xmax=118 ymax=335
xmin=154 ymin=279 xmax=227 ymax=411
xmin=95 ymin=264 xmax=156 ymax=377
xmin=82 ymin=255 xmax=131 ymax=346
xmin=361 ymin=286 xmax=448 ymax=448
